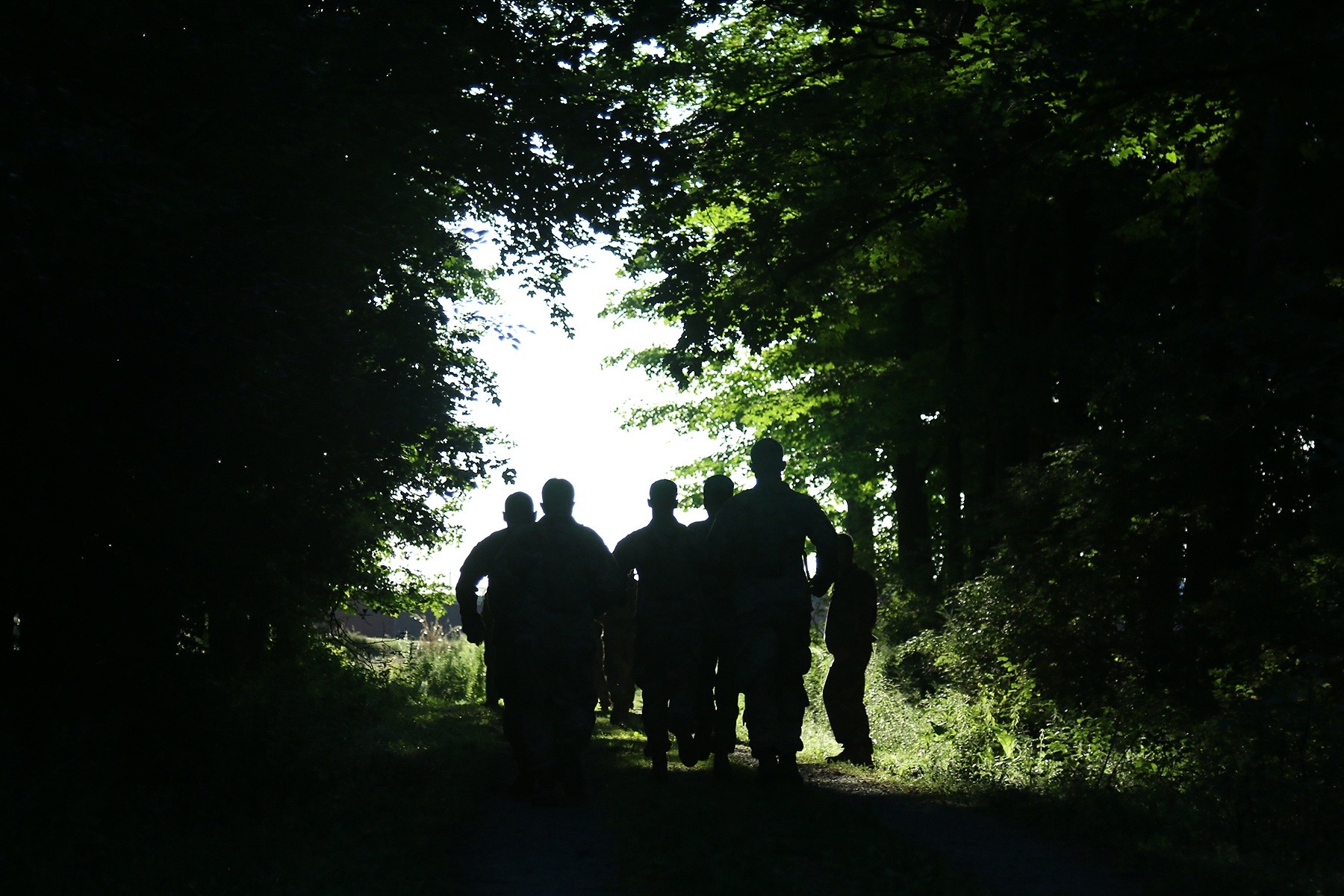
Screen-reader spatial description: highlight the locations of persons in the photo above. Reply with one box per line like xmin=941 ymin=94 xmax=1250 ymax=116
xmin=456 ymin=438 xmax=878 ymax=804
